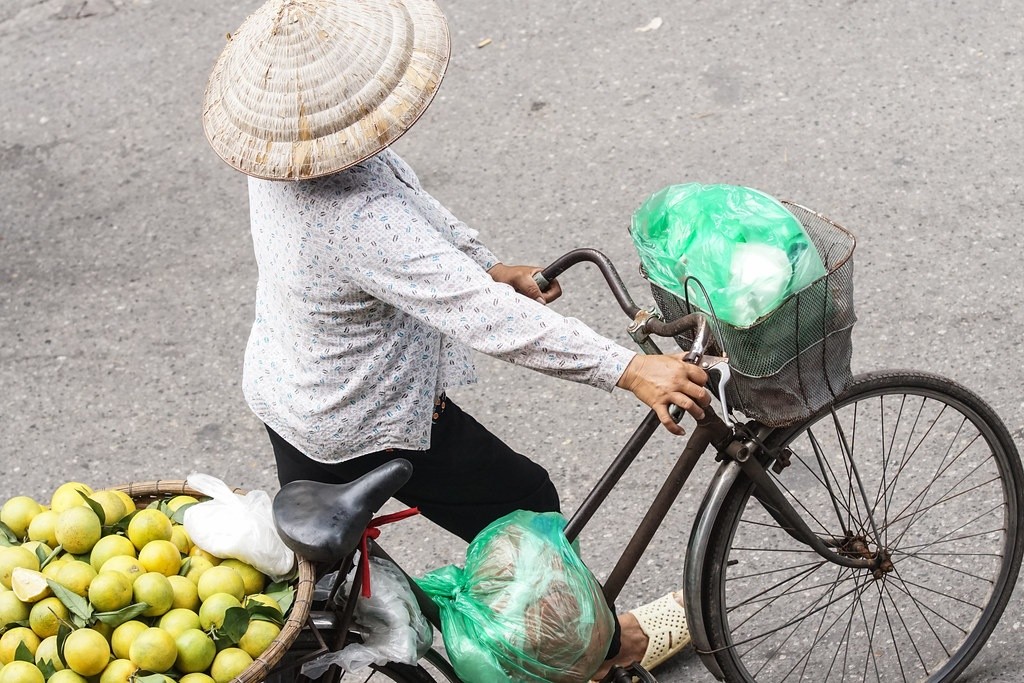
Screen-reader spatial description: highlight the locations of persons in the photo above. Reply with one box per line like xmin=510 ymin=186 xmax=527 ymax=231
xmin=202 ymin=0 xmax=711 ymax=683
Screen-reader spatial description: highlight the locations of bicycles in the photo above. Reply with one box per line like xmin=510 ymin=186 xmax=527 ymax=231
xmin=258 ymin=194 xmax=1024 ymax=683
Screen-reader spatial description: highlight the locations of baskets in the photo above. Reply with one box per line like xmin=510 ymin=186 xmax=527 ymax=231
xmin=639 ymin=202 xmax=856 ymax=428
xmin=46 ymin=480 xmax=315 ymax=683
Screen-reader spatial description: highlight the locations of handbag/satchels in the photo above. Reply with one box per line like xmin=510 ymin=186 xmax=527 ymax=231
xmin=630 ymin=183 xmax=833 ymax=374
xmin=410 ymin=509 xmax=615 ymax=683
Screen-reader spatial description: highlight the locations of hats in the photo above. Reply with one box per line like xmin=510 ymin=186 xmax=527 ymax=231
xmin=203 ymin=0 xmax=451 ymax=180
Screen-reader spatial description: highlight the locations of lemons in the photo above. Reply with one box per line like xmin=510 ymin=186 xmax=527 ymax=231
xmin=0 ymin=481 xmax=283 ymax=683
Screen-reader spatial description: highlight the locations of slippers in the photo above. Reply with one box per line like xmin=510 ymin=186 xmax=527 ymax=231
xmin=588 ymin=589 xmax=691 ymax=683
